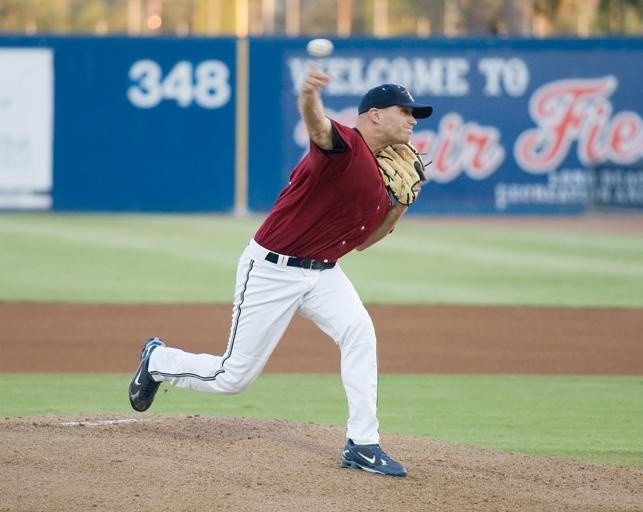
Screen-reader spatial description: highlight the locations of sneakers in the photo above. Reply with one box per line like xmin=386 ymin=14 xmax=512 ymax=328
xmin=128 ymin=337 xmax=167 ymax=413
xmin=338 ymin=438 xmax=408 ymax=478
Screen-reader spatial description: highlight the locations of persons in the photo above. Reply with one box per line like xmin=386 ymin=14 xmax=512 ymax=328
xmin=126 ymin=62 xmax=432 ymax=477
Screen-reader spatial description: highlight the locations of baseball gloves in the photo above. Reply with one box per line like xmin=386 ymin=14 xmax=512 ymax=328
xmin=373 ymin=142 xmax=432 ymax=207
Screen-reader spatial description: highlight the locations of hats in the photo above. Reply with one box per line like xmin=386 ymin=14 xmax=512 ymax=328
xmin=357 ymin=83 xmax=434 ymax=120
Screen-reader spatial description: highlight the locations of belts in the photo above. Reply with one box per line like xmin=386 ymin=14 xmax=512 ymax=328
xmin=246 ymin=241 xmax=336 ymax=271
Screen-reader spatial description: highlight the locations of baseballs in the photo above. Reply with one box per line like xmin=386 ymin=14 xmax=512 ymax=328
xmin=307 ymin=38 xmax=332 ymax=56
